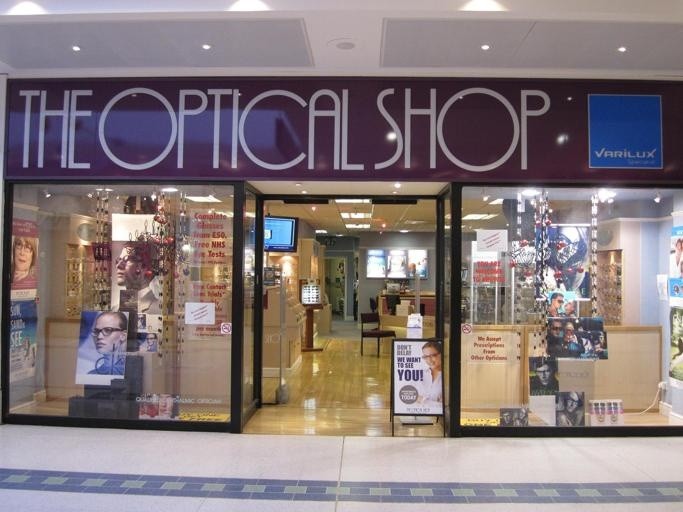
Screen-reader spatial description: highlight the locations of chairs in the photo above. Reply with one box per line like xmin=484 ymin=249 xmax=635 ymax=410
xmin=358 ymin=311 xmax=396 ymax=358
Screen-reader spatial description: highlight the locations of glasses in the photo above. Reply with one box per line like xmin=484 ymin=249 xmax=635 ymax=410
xmin=565 ymin=396 xmax=579 ymax=406
xmin=91 ymin=325 xmax=125 ymax=338
xmin=535 ymin=370 xmax=551 ymax=375
xmin=13 ymin=241 xmax=33 ymax=253
xmin=115 ymin=255 xmax=142 ymax=264
xmin=146 ymin=337 xmax=154 ymax=340
xmin=138 ymin=317 xmax=143 ymax=320
xmin=421 ymin=352 xmax=440 ymax=361
xmin=554 ymin=299 xmax=564 ymax=303
xmin=550 ymin=326 xmax=563 ymax=331
xmin=304 ymin=286 xmax=319 ymax=303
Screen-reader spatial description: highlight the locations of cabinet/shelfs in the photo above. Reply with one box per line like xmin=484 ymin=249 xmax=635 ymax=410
xmin=49 ymin=214 xmax=109 ymax=315
xmin=591 ymin=249 xmax=639 ymax=324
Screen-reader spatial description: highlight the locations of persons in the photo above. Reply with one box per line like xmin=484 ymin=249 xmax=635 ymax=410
xmin=413 ymin=342 xmax=442 ymax=406
xmin=530 ymin=357 xmax=557 ymax=395
xmin=20 ymin=334 xmax=32 ymax=358
xmin=548 ymin=291 xmax=607 ymax=358
xmin=116 ymin=243 xmax=160 ymax=313
xmin=12 ymin=237 xmax=37 ymax=283
xmin=501 ymin=409 xmax=527 ymax=427
xmin=557 ymin=392 xmax=586 ymax=426
xmin=668 ymin=238 xmax=683 ymax=278
xmin=87 ymin=312 xmax=127 ymax=375
xmin=143 ymin=333 xmax=158 ymax=353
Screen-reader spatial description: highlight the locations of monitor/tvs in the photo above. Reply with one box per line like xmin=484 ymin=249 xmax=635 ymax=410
xmin=250 ymin=215 xmax=299 ymax=253
